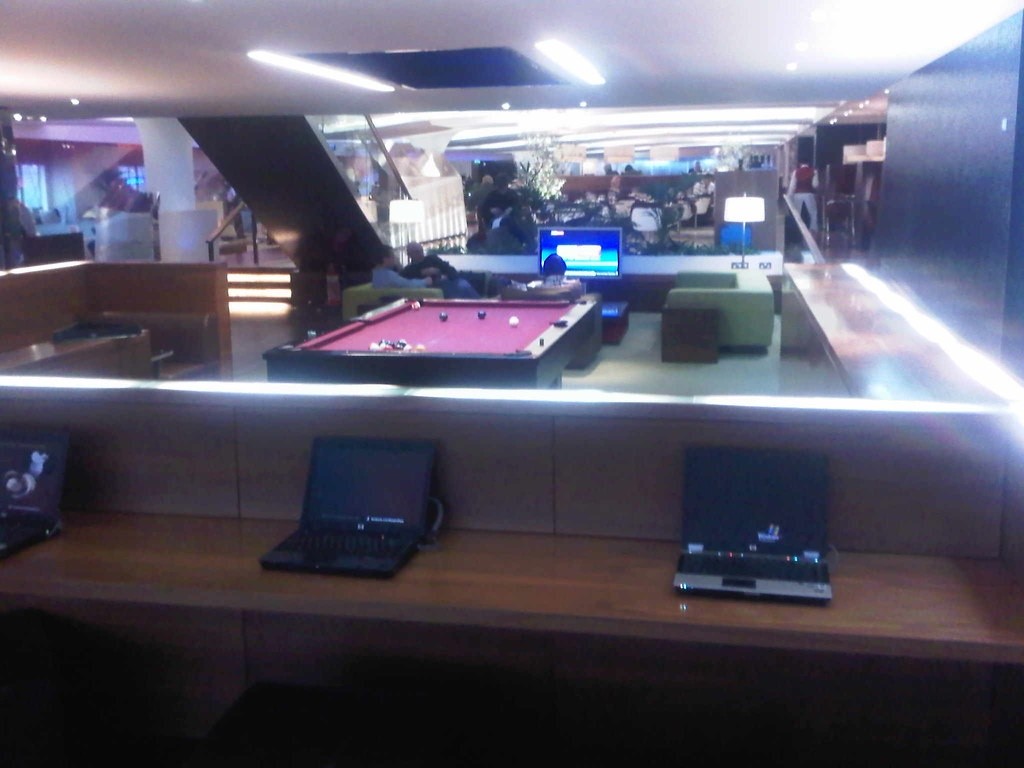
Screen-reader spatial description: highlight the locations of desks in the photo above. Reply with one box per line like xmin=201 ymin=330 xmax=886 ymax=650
xmin=264 ymin=298 xmax=596 ymax=391
xmin=0 ymin=370 xmax=1024 ymax=768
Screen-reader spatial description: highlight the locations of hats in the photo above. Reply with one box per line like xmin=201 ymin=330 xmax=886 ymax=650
xmin=544 ymin=254 xmax=566 ymax=273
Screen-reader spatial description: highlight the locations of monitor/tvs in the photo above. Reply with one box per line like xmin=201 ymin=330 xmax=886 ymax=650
xmin=537 ymin=227 xmax=623 ymax=282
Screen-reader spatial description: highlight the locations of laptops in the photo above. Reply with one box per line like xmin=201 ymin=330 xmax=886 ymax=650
xmin=673 ymin=445 xmax=832 ymax=602
xmin=262 ymin=435 xmax=438 ymax=579
xmin=0 ymin=427 xmax=72 ymax=556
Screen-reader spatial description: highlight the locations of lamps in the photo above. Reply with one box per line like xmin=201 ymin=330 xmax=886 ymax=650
xmin=723 ymin=191 xmax=764 ymax=268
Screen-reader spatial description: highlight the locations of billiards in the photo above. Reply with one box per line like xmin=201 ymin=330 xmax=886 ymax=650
xmin=478 ymin=310 xmax=486 ymax=319
xmin=509 ymin=315 xmax=519 ymax=326
xmin=412 ymin=301 xmax=420 ymax=311
xmin=439 ymin=311 xmax=448 ymax=321
xmin=368 ymin=339 xmax=426 ymax=352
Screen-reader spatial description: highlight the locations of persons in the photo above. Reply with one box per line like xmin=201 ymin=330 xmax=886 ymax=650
xmin=604 ymin=163 xmax=640 ymax=175
xmin=372 ymin=245 xmax=432 ymax=289
xmin=398 ymin=242 xmax=482 ymax=299
xmin=607 ymin=176 xmax=631 ymax=200
xmin=529 ymin=254 xmax=580 ymax=288
xmin=86 ymin=170 xmax=139 ymax=256
xmin=789 ymin=157 xmax=819 ymax=233
xmin=693 ymin=173 xmax=715 ymax=196
xmin=467 ymin=174 xmax=538 ymax=254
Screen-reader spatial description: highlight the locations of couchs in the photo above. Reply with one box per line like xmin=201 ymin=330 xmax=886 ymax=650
xmin=660 ymin=269 xmax=775 ymax=363
xmin=343 ymin=271 xmax=493 ymax=327
xmin=494 ymin=286 xmax=602 ymax=369
